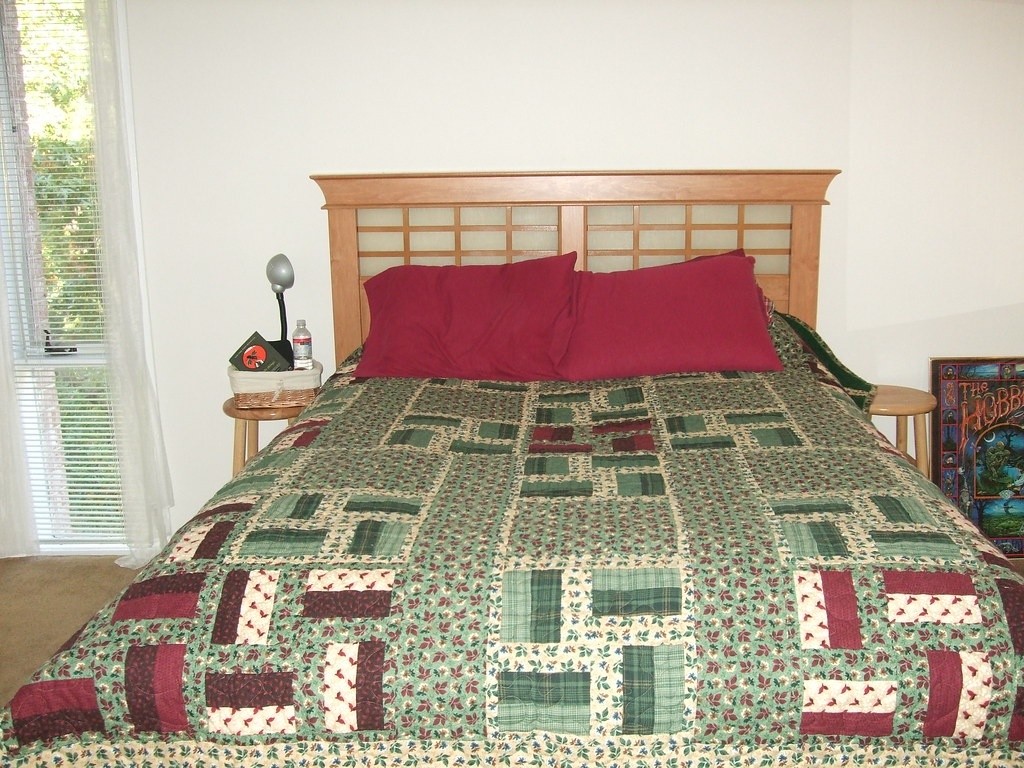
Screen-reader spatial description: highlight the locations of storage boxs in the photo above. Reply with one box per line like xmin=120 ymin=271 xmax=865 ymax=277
xmin=228 ymin=358 xmax=322 ymax=393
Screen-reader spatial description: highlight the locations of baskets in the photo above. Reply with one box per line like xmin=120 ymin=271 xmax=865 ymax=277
xmin=226 ymin=359 xmax=323 ymax=410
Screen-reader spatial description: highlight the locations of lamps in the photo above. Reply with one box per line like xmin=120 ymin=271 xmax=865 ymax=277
xmin=266 ymin=254 xmax=294 ymax=342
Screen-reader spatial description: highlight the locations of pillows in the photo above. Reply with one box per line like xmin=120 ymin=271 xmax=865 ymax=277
xmin=561 ymin=251 xmax=779 ymax=377
xmin=349 ymin=254 xmax=576 ymax=383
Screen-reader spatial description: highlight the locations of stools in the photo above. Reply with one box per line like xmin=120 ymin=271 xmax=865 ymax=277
xmin=222 ymin=396 xmax=304 ymax=477
xmin=869 ymin=385 xmax=937 ymax=477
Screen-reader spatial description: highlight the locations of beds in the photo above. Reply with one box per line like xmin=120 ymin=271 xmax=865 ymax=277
xmin=0 ymin=169 xmax=1023 ymax=768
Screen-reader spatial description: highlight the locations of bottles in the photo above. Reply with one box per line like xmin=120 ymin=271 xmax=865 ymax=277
xmin=292 ymin=320 xmax=313 ymax=371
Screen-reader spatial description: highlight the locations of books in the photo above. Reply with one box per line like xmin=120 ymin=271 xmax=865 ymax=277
xmin=229 ymin=331 xmax=294 ymax=372
